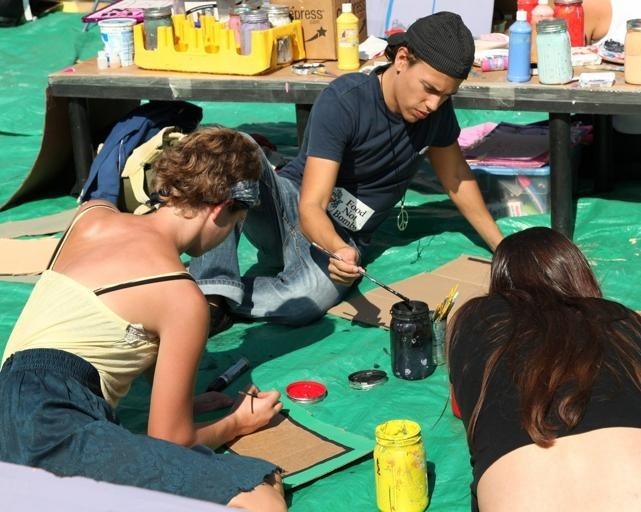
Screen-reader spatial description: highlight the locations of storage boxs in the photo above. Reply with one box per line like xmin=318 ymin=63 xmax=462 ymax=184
xmin=270 ymin=0 xmax=366 ymax=60
xmin=469 ymin=132 xmax=593 ymax=217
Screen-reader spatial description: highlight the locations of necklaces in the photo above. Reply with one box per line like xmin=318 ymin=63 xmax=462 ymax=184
xmin=382 ymin=72 xmax=412 ymax=232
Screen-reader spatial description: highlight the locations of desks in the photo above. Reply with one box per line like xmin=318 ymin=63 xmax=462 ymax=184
xmin=48 ymin=55 xmax=641 ymax=242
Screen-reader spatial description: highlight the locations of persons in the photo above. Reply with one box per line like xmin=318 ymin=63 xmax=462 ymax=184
xmin=526 ymin=0 xmax=641 ymax=199
xmin=188 ymin=11 xmax=508 ymax=339
xmin=441 ymin=224 xmax=640 ymax=512
xmin=0 ymin=127 xmax=291 ymax=510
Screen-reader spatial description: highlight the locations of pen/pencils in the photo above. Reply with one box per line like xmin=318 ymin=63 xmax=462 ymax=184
xmin=468 ymin=257 xmax=492 ymax=266
xmin=238 ymin=391 xmax=258 ymax=397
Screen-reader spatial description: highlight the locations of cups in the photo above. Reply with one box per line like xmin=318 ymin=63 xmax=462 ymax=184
xmin=427 ymin=310 xmax=447 ymax=366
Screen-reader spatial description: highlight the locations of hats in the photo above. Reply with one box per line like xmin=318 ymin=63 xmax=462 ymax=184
xmin=387 ymin=9 xmax=476 ymax=81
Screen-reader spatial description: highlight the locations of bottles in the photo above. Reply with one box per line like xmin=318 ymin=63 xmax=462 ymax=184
xmin=372 ymin=419 xmax=430 ymax=512
xmin=623 ymin=19 xmax=641 ymax=85
xmin=142 ymin=9 xmax=177 ymax=51
xmin=96 ymin=51 xmax=107 ymax=70
xmin=553 ymin=0 xmax=585 ymax=47
xmin=108 ymin=48 xmax=122 ymax=69
xmin=390 ymin=299 xmax=436 ymax=382
xmin=480 ymin=58 xmax=508 ymax=72
xmin=531 ymin=0 xmax=555 ymax=30
xmin=535 ymin=18 xmax=573 ymax=84
xmin=516 ymin=0 xmax=538 ymax=24
xmin=507 ymin=9 xmax=532 ymax=82
xmin=227 ymin=4 xmax=294 ymax=64
xmin=334 ymin=3 xmax=360 ymax=72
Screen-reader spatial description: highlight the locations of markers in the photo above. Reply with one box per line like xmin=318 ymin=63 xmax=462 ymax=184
xmin=207 ymin=356 xmax=251 ymax=391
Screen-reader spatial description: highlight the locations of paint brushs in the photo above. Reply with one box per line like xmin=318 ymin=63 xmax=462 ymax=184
xmin=309 ymin=240 xmax=415 ymax=310
